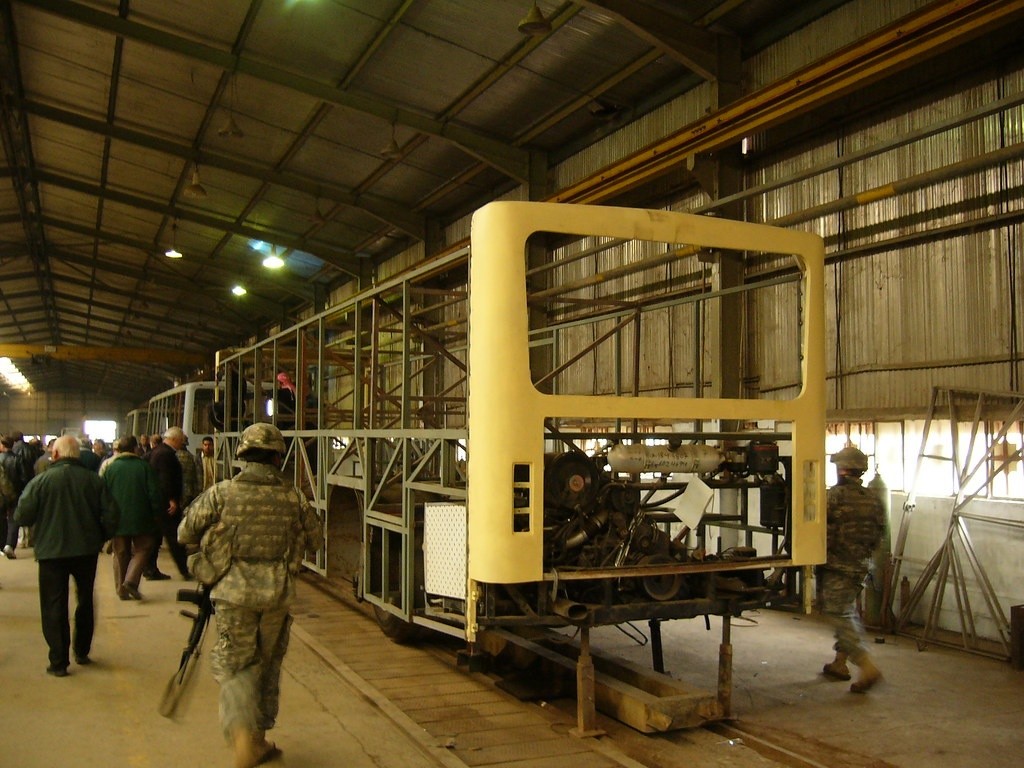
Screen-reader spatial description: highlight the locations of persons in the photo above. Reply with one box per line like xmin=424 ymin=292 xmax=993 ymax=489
xmin=814 ymin=446 xmax=891 ymax=692
xmin=0 ymin=426 xmax=221 ymax=677
xmin=178 ymin=423 xmax=317 ymax=768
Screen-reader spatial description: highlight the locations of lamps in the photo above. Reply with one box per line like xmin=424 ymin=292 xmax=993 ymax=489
xmin=262 ymin=245 xmax=285 ymax=270
xmin=381 ymin=124 xmax=402 ymax=159
xmin=308 ymin=198 xmax=324 ymax=222
xmin=219 ymin=73 xmax=244 ymax=139
xmin=183 ymin=164 xmax=208 ymax=199
xmin=165 ymin=218 xmax=183 ymax=258
xmin=518 ymin=0 xmax=552 ymax=36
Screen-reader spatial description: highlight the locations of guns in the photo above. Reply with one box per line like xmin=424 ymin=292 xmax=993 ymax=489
xmin=175 ymin=581 xmax=219 ymax=685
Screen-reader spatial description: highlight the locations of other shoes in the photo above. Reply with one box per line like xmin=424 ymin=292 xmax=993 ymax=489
xmin=0 ymin=544 xmax=17 ymax=559
xmin=77 ymin=653 xmax=91 ymax=664
xmin=120 ymin=593 xmax=129 ymax=601
xmin=123 ymin=581 xmax=142 ymax=600
xmin=225 ymin=718 xmax=278 ymax=767
xmin=145 ymin=572 xmax=171 ymax=580
xmin=182 ymin=571 xmax=195 ymax=581
xmin=46 ymin=663 xmax=68 ymax=677
xmin=850 ymin=653 xmax=880 ymax=693
xmin=824 ymin=650 xmax=851 ymax=681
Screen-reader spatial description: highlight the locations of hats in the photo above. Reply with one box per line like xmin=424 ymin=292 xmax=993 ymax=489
xmin=182 ymin=436 xmax=189 ymax=446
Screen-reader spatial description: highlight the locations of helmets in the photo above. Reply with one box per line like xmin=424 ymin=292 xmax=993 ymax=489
xmin=830 ymin=448 xmax=868 ymax=470
xmin=235 ymin=422 xmax=286 ymax=457
